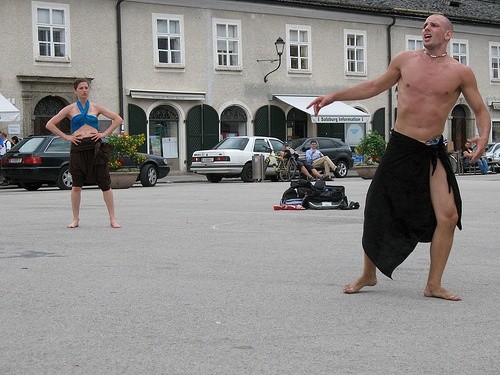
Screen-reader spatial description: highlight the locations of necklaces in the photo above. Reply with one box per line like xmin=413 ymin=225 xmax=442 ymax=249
xmin=422 ymin=47 xmax=447 ymax=59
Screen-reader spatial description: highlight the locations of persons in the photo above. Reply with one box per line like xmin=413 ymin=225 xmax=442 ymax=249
xmin=45 ymin=79 xmax=123 ymax=228
xmin=306 ymin=140 xmax=342 ymax=181
xmin=276 ymin=147 xmax=329 ymax=182
xmin=0 ymin=129 xmax=20 ymax=186
xmin=306 ymin=14 xmax=491 ymax=301
xmin=462 ymin=141 xmax=492 ymax=175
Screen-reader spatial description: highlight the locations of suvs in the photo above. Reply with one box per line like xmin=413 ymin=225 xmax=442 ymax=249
xmin=284 ymin=137 xmax=355 ymax=178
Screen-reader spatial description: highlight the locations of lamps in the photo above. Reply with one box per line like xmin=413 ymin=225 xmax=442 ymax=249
xmin=256 ymin=37 xmax=286 ymax=83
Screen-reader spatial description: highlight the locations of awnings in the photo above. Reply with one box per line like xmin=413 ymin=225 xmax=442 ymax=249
xmin=0 ymin=94 xmax=20 ymax=121
xmin=273 ymin=95 xmax=371 ymax=123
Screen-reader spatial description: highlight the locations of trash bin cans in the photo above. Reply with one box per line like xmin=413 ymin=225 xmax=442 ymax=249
xmin=252 ymin=154 xmax=265 ymax=182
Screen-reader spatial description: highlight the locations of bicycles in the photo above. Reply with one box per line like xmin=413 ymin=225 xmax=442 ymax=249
xmin=258 ymin=145 xmax=298 ymax=182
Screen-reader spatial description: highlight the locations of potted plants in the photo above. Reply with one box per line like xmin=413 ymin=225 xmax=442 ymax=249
xmin=353 ymin=130 xmax=388 ymax=179
xmin=104 ymin=133 xmax=147 ymax=188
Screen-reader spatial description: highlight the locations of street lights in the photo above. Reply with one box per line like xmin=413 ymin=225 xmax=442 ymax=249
xmin=257 ymin=36 xmax=285 ymax=83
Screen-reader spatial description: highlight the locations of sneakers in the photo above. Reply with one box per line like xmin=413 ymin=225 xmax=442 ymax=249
xmin=484 ymin=170 xmax=493 ymax=174
xmin=309 ymin=167 xmax=341 ymax=181
xmin=0 ymin=180 xmax=8 ymax=186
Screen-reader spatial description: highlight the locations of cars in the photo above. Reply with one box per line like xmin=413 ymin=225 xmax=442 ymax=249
xmin=471 ymin=142 xmax=495 ymax=161
xmin=0 ymin=132 xmax=170 ymax=191
xmin=188 ymin=135 xmax=299 ymax=183
xmin=484 ymin=142 xmax=500 ymax=173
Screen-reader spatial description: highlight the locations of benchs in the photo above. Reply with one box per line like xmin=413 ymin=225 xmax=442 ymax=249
xmin=299 ymin=151 xmax=334 ymax=183
xmin=453 ymin=150 xmax=500 ymax=176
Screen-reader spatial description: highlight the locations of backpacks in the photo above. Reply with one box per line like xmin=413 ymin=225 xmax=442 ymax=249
xmin=5 ymin=140 xmax=14 ymax=151
